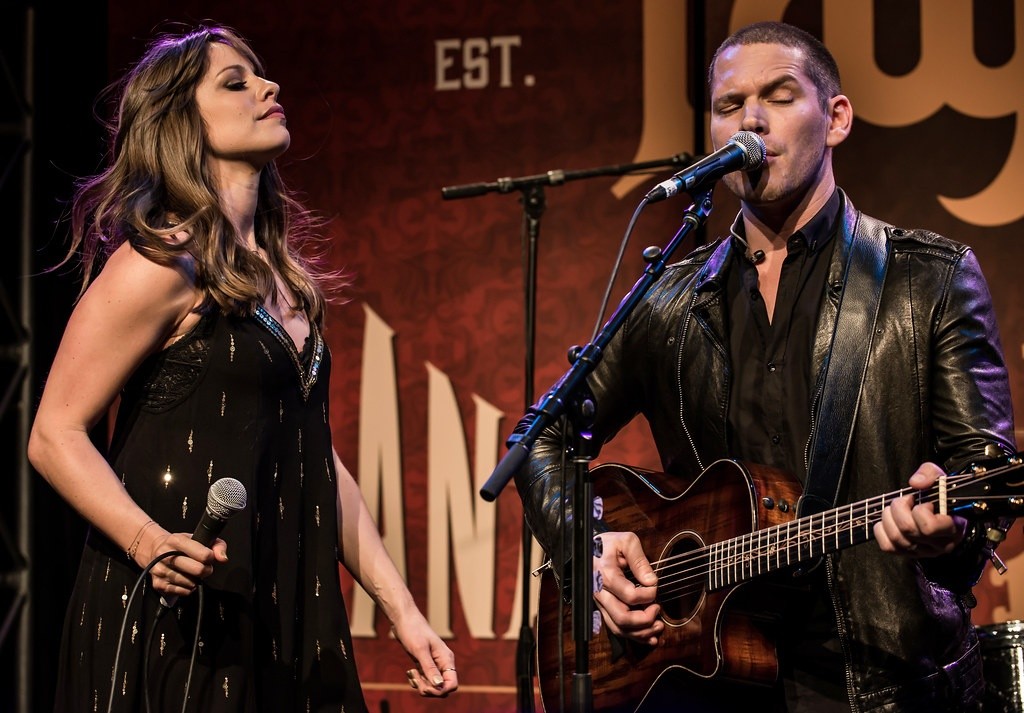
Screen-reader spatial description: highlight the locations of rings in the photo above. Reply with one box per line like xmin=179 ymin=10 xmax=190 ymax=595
xmin=442 ymin=668 xmax=457 ymax=673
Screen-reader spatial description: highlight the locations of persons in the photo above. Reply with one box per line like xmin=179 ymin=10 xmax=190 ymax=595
xmin=24 ymin=17 xmax=459 ymax=713
xmin=507 ymin=21 xmax=1017 ymax=713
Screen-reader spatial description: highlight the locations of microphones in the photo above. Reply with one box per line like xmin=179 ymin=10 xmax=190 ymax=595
xmin=644 ymin=130 xmax=767 ymax=205
xmin=155 ymin=477 xmax=248 ymax=620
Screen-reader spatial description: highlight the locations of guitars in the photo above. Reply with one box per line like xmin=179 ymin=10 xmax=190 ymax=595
xmin=537 ymin=453 xmax=1024 ymax=713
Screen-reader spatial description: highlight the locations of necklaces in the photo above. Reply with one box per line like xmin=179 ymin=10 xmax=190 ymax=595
xmin=164 ymin=221 xmax=263 ymax=260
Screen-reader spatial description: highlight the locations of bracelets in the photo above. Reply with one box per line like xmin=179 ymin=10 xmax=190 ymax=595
xmin=131 ymin=522 xmax=159 ymax=560
xmin=126 ymin=519 xmax=154 ymax=560
